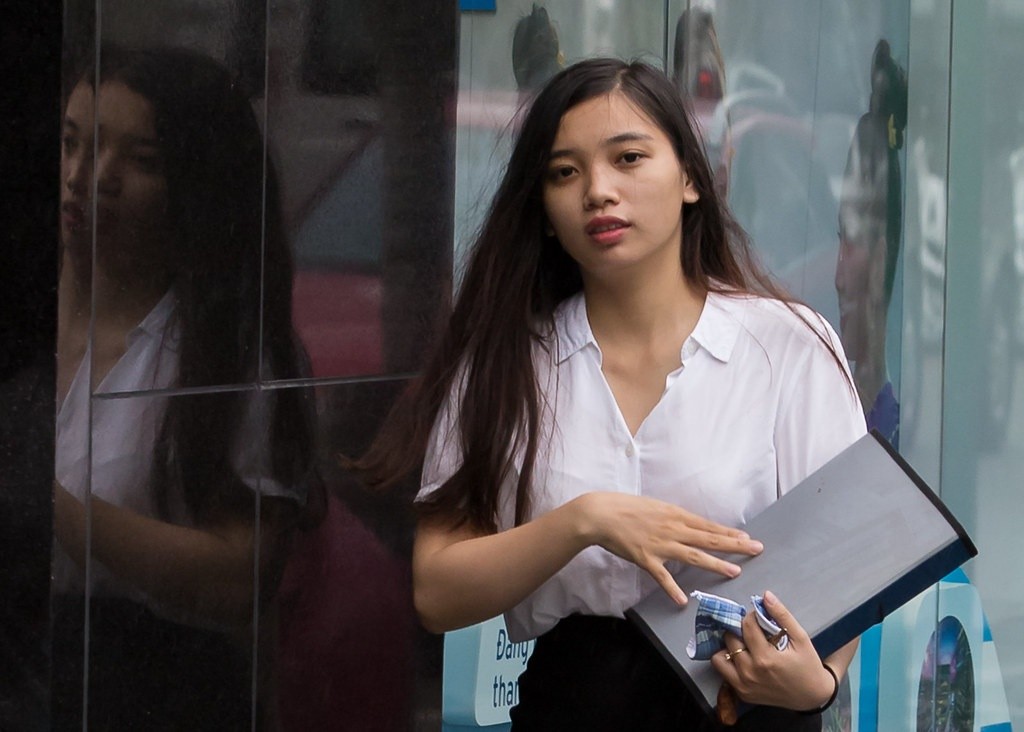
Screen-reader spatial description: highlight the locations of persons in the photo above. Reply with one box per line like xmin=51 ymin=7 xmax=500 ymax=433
xmin=47 ymin=49 xmax=319 ymax=731
xmin=340 ymin=55 xmax=869 ymax=731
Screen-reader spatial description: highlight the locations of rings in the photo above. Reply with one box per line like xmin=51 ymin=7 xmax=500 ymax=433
xmin=725 ymin=648 xmax=745 ymax=661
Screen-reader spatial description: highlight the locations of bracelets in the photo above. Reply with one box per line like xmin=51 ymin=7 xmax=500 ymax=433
xmin=796 ymin=664 xmax=839 ymax=716
